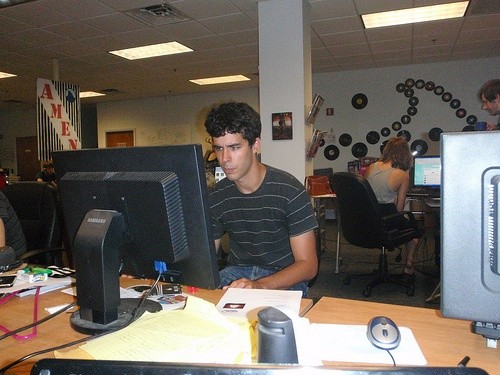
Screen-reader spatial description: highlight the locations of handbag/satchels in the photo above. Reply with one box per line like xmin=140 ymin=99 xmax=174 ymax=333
xmin=304 ymin=175 xmax=336 ymax=197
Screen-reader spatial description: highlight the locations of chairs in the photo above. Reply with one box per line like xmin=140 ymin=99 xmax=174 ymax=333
xmin=329 ymin=170 xmax=420 ymax=298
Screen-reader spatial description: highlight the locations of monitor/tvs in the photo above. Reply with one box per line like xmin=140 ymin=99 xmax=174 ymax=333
xmin=410 ymin=130 xmax=500 ymax=325
xmin=212 ymin=164 xmax=227 ymax=183
xmin=50 ymin=143 xmax=222 ymax=334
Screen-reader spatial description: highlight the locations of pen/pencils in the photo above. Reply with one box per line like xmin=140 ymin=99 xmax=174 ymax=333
xmin=459 ymin=356 xmax=470 ymax=368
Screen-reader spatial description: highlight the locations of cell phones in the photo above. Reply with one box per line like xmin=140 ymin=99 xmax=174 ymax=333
xmin=0 ymin=275 xmax=17 ymax=288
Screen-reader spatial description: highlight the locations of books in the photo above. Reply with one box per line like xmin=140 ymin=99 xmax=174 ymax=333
xmin=0 ymin=266 xmax=77 ymax=297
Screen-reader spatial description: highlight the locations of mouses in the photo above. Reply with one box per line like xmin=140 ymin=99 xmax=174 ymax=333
xmin=366 ymin=316 xmax=401 ymax=350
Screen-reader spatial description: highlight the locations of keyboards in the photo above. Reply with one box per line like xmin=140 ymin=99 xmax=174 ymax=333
xmin=471 ymin=321 xmax=500 ymax=340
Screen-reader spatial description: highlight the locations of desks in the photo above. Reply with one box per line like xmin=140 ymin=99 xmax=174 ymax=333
xmin=311 ymin=189 xmax=434 ymax=275
xmin=0 ymin=261 xmax=500 ymax=375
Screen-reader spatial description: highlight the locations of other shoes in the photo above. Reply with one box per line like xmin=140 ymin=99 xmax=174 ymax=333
xmin=399 ymin=268 xmax=416 ymax=294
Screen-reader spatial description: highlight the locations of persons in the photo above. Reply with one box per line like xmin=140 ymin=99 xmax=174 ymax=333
xmin=476 ymin=77 xmax=500 ymax=133
xmin=363 ymin=136 xmax=422 ymax=277
xmin=205 ymin=102 xmax=320 ymax=297
xmin=0 ymin=190 xmax=28 ymax=268
xmin=34 ymin=160 xmax=58 ymax=189
